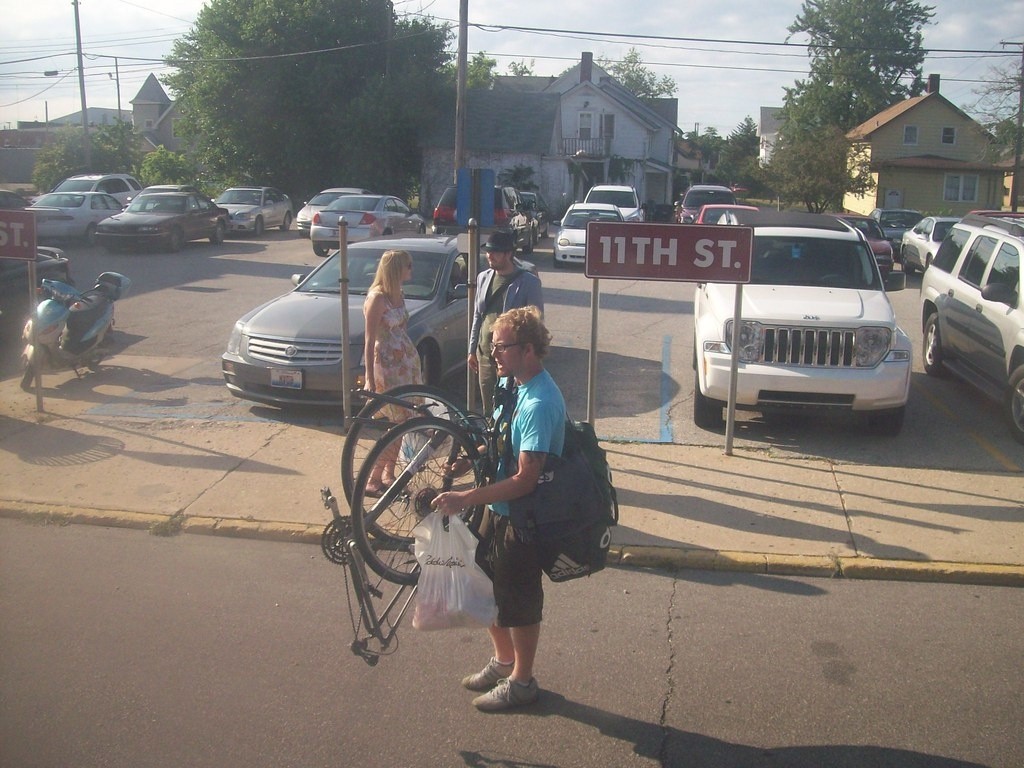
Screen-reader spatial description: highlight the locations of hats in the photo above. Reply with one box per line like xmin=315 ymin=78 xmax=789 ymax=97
xmin=480 ymin=230 xmax=515 ymax=252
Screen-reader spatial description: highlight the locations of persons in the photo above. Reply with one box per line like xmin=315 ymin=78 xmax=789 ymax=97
xmin=468 ymin=230 xmax=544 ymax=419
xmin=363 ymin=248 xmax=425 ymax=498
xmin=646 ymin=194 xmax=657 ymax=222
xmin=432 ymin=308 xmax=567 ymax=712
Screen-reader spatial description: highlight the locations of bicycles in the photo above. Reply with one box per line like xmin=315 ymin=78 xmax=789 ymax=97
xmin=319 ymin=383 xmax=498 ymax=667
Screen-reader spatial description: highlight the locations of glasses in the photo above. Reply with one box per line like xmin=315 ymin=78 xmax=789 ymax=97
xmin=489 ymin=342 xmax=519 ymax=353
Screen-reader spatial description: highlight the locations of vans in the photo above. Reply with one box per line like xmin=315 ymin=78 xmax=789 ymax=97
xmin=674 ymin=184 xmax=735 ymax=226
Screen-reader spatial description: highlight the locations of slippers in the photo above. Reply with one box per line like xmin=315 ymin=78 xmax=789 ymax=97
xmin=364 ymin=477 xmax=412 ymax=501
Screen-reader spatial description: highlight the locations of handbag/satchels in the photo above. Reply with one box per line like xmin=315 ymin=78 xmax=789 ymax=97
xmin=497 ymin=387 xmax=619 ymax=583
xmin=406 ymin=509 xmax=499 ymax=631
xmin=401 ymin=398 xmax=453 ymax=461
xmin=353 ymin=416 xmax=390 ymax=442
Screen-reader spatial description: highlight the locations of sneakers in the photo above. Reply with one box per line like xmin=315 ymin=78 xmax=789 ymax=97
xmin=473 ymin=677 xmax=539 ymax=713
xmin=462 ymin=657 xmax=517 ymax=691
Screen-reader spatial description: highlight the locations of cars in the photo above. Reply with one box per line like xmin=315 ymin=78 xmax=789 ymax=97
xmin=124 ymin=184 xmax=209 ymax=211
xmin=805 ymin=212 xmax=894 ymax=281
xmin=689 ymin=203 xmax=759 ymax=227
xmin=24 ymin=191 xmax=127 ymax=247
xmin=520 ymin=191 xmax=550 ymax=245
xmin=552 ymin=202 xmax=625 ymax=269
xmin=0 ymin=246 xmax=76 ymax=325
xmin=869 ymin=208 xmax=925 ymax=262
xmin=94 ymin=191 xmax=230 ymax=254
xmin=220 ymin=233 xmax=539 ymax=413
xmin=308 ymin=192 xmax=427 ymax=257
xmin=296 ymin=187 xmax=375 ymax=237
xmin=0 ymin=189 xmax=33 ymax=210
xmin=900 ymin=216 xmax=964 ymax=274
xmin=210 ymin=185 xmax=294 ymax=236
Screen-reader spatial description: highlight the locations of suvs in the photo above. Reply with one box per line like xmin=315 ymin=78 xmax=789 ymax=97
xmin=917 ymin=209 xmax=1023 ymax=448
xmin=29 ymin=173 xmax=143 ymax=206
xmin=431 ymin=183 xmax=537 ymax=255
xmin=575 ymin=183 xmax=647 ymax=223
xmin=690 ymin=207 xmax=913 ymax=437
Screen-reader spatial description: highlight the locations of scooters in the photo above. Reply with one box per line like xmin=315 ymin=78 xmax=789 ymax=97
xmin=19 ymin=271 xmax=134 ymax=390
